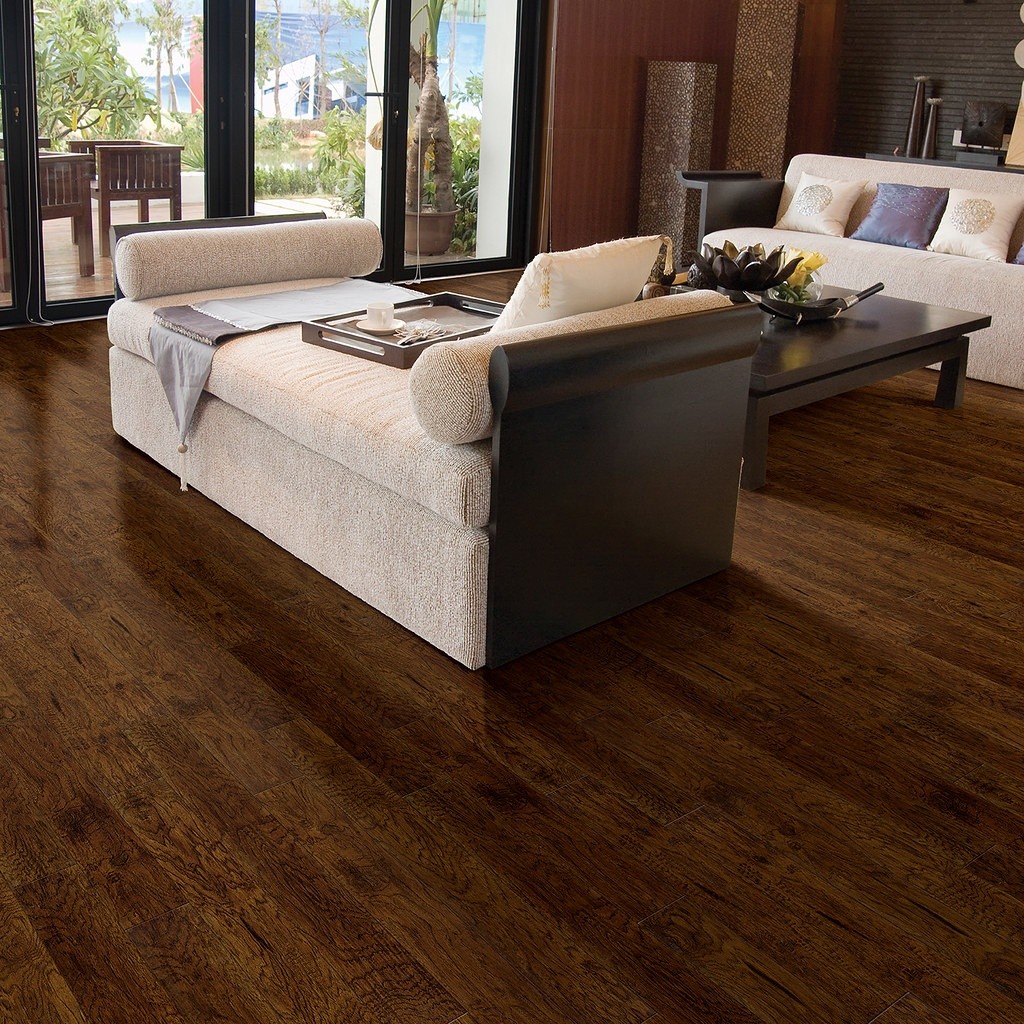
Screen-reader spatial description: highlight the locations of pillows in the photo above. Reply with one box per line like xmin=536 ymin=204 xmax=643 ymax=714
xmin=773 ymin=170 xmax=870 ymax=236
xmin=489 ymin=235 xmax=664 ymax=333
xmin=848 ymin=182 xmax=950 ymax=256
xmin=925 ymin=187 xmax=1023 ymax=264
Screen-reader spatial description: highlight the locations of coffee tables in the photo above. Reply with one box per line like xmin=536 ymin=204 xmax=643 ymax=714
xmin=647 ymin=269 xmax=995 ymax=492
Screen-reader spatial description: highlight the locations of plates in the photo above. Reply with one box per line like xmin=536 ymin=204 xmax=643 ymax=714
xmin=356 ymin=319 xmax=405 ymax=336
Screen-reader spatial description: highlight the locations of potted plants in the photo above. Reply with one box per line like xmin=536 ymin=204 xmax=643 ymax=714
xmin=401 ymin=1 xmax=462 ymax=257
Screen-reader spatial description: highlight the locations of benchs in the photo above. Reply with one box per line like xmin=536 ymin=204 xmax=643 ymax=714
xmin=106 ymin=219 xmax=760 ymax=675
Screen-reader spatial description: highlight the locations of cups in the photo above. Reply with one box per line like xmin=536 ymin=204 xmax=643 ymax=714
xmin=366 ymin=301 xmax=394 ymax=329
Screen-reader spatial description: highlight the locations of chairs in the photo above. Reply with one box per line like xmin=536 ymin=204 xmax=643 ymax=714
xmin=0 ymin=135 xmax=95 ymax=294
xmin=69 ymin=139 xmax=184 ymax=259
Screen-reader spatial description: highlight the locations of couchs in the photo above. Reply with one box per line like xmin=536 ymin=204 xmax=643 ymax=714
xmin=704 ymin=152 xmax=1024 ymax=389
